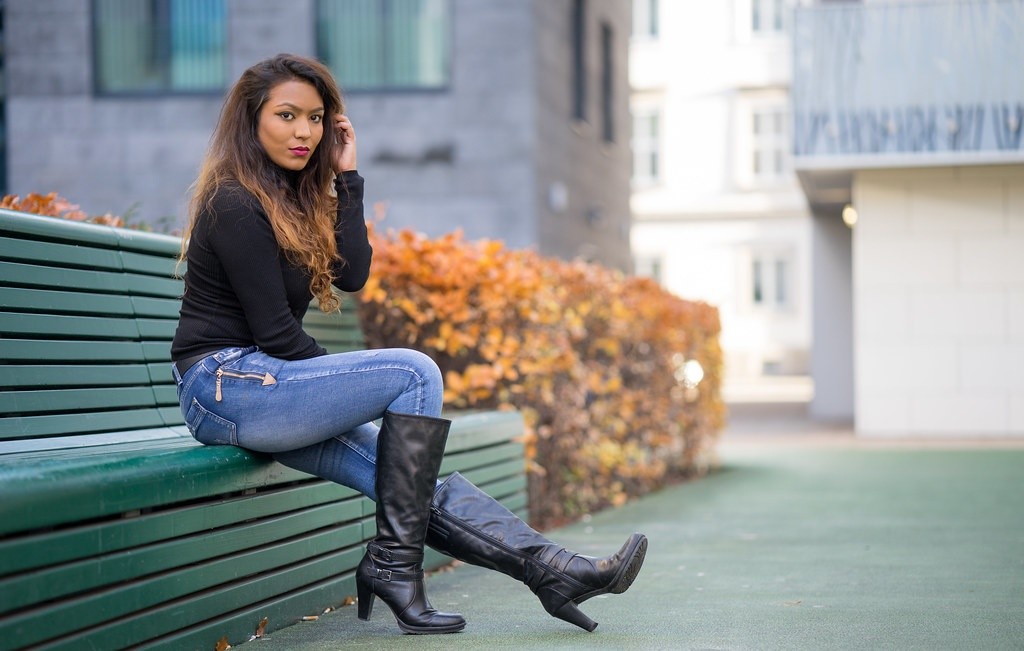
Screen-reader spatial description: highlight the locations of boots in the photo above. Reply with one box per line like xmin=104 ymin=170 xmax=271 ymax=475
xmin=423 ymin=471 xmax=649 ymax=632
xmin=356 ymin=413 xmax=466 ymax=635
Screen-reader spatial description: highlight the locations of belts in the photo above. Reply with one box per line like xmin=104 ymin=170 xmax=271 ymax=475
xmin=172 ymin=347 xmax=225 ymax=387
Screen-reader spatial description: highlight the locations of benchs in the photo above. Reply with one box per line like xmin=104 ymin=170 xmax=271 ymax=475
xmin=0 ymin=209 xmax=531 ymax=651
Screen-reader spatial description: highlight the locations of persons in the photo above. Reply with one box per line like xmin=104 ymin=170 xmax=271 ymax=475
xmin=169 ymin=53 xmax=648 ymax=634
